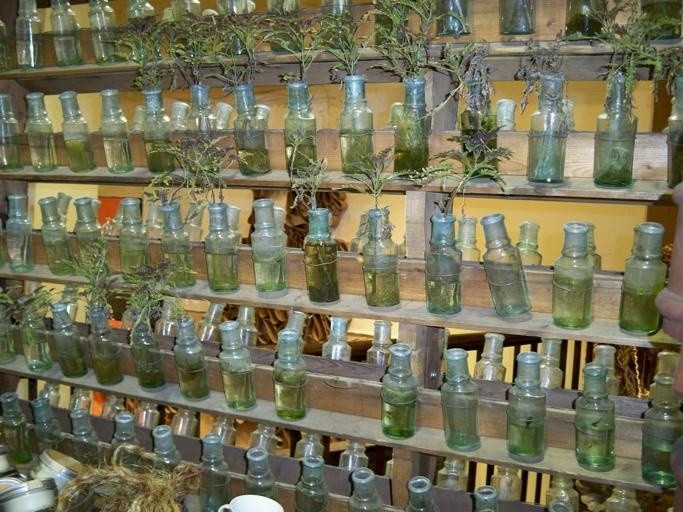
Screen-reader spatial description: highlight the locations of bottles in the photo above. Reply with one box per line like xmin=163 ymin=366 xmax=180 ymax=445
xmin=3 ymin=193 xmax=680 ymax=325
xmin=3 ymin=300 xmax=673 ymax=511
xmin=0 ymin=67 xmax=680 ymax=190
xmin=8 ymin=0 xmax=683 ymax=65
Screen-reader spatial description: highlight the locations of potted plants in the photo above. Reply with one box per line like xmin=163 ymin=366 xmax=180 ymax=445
xmin=0 ymin=232 xmax=211 ymax=400
xmin=103 ymin=6 xmax=680 ymax=309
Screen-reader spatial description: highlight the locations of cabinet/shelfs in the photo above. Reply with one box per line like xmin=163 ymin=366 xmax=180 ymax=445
xmin=0 ymin=0 xmax=682 ymax=512
